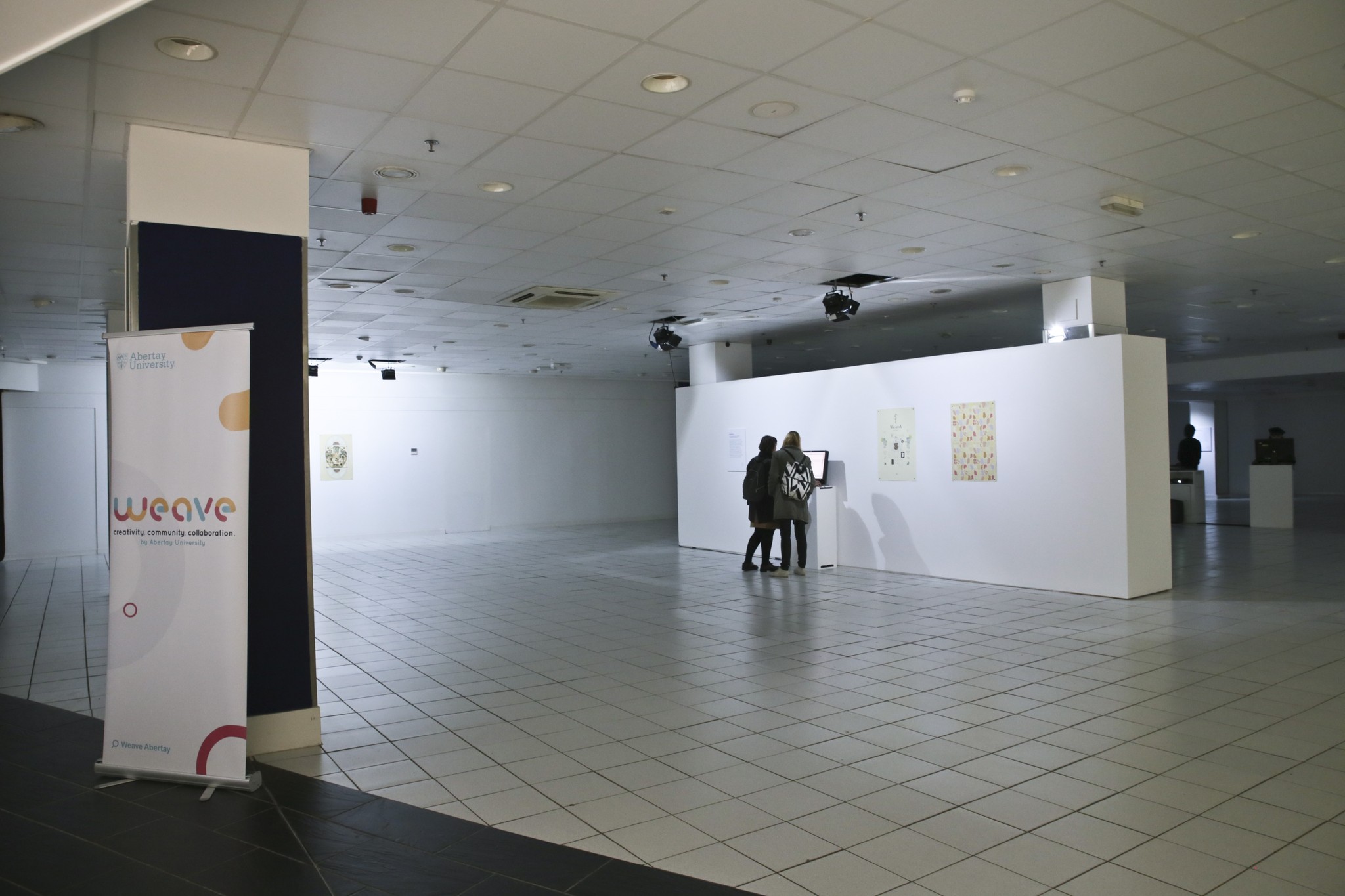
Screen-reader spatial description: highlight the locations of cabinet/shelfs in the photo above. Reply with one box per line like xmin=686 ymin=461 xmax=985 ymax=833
xmin=1170 ymin=470 xmax=1205 ymax=523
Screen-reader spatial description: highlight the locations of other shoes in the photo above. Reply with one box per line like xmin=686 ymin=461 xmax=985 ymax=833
xmin=769 ymin=568 xmax=789 ymax=577
xmin=794 ymin=566 xmax=805 ymax=575
xmin=742 ymin=562 xmax=758 ymax=570
xmin=760 ymin=563 xmax=781 ymax=572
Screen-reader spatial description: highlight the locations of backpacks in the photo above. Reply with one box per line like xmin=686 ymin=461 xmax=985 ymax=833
xmin=742 ymin=455 xmax=774 ymax=502
xmin=780 ymin=447 xmax=812 ymax=500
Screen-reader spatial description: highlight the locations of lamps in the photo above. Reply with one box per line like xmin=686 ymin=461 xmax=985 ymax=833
xmin=1100 ymin=195 xmax=1144 ymax=218
xmin=1201 ymin=336 xmax=1220 ymax=343
xmin=647 ymin=320 xmax=682 ymax=352
xmin=309 ymin=364 xmax=319 ymax=377
xmin=381 ymin=368 xmax=395 ymax=381
xmin=822 ymin=278 xmax=860 ymax=323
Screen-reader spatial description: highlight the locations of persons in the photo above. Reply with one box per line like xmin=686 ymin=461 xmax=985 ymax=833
xmin=766 ymin=431 xmax=818 ymax=578
xmin=741 ymin=435 xmax=822 ymax=573
xmin=1254 ymin=427 xmax=1295 ymax=465
xmin=1174 ymin=423 xmax=1202 ymax=471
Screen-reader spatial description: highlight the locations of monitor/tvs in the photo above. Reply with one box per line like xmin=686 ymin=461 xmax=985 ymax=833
xmin=1255 ymin=438 xmax=1295 ymax=464
xmin=802 ymin=451 xmax=829 ymax=483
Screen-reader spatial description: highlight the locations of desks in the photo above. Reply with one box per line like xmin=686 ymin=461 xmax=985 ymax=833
xmin=789 ymin=485 xmax=837 ymax=569
xmin=1249 ymin=464 xmax=1293 ymax=529
